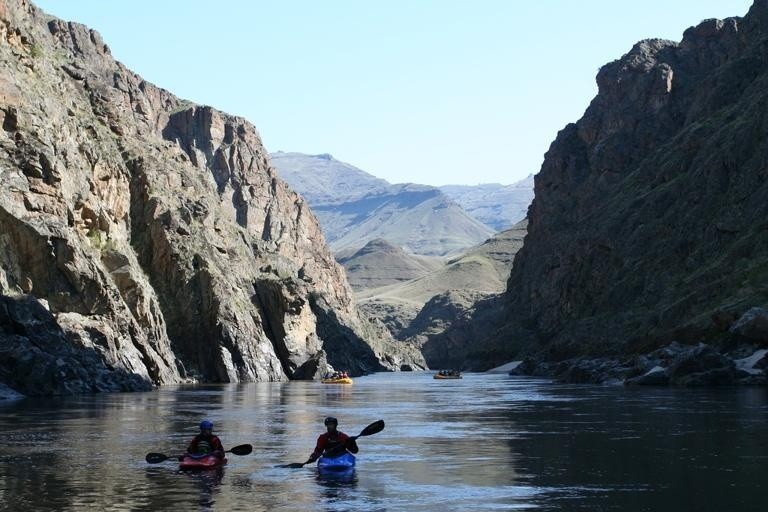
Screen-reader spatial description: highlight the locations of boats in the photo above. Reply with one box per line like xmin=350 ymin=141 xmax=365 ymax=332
xmin=432 ymin=373 xmax=463 ymax=381
xmin=179 ymin=454 xmax=229 ymax=471
xmin=321 ymin=377 xmax=353 ymax=385
xmin=316 ymin=449 xmax=357 ymax=484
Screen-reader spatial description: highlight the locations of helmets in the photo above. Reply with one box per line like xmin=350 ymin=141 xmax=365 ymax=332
xmin=200 ymin=421 xmax=213 ymax=429
xmin=325 ymin=417 xmax=338 ymax=426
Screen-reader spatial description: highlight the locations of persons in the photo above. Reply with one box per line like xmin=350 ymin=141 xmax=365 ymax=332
xmin=439 ymin=368 xmax=460 ymax=376
xmin=322 ymin=370 xmax=348 ymax=379
xmin=306 ymin=417 xmax=358 ymax=464
xmin=184 ymin=420 xmax=225 ymax=458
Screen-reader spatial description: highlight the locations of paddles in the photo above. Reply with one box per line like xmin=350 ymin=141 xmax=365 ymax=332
xmin=146 ymin=442 xmax=252 ymax=464
xmin=288 ymin=420 xmax=384 ymax=468
